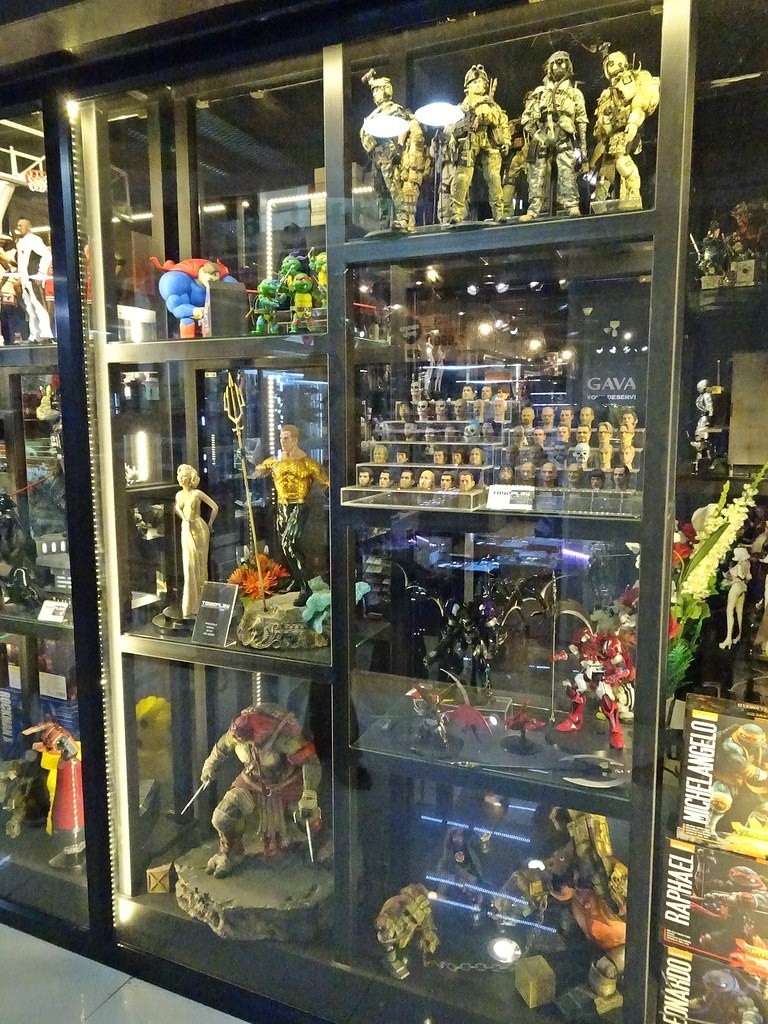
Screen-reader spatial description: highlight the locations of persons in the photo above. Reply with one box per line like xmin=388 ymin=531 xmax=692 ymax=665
xmin=359 ymin=382 xmax=638 ymax=494
xmin=694 ymin=380 xmax=714 ymax=463
xmin=0 ymin=216 xmax=90 ymax=874
xmin=359 ymin=49 xmax=661 ymax=233
xmin=373 ymin=570 xmax=638 ymax=1016
xmin=172 ymin=421 xmax=333 ymax=878
xmin=699 ymin=201 xmax=768 ymax=276
xmin=150 ymin=253 xmax=330 ymax=340
xmin=719 ymin=546 xmax=752 ymax=649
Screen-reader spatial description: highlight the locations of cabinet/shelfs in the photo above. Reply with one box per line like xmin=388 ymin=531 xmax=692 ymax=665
xmin=0 ymin=0 xmax=766 ymax=1022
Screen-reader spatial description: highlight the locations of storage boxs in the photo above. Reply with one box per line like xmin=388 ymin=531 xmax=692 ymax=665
xmin=654 ymin=693 xmax=767 ymax=1023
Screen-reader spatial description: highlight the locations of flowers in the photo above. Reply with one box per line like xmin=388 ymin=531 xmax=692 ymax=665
xmin=589 ymin=465 xmax=768 ymax=699
